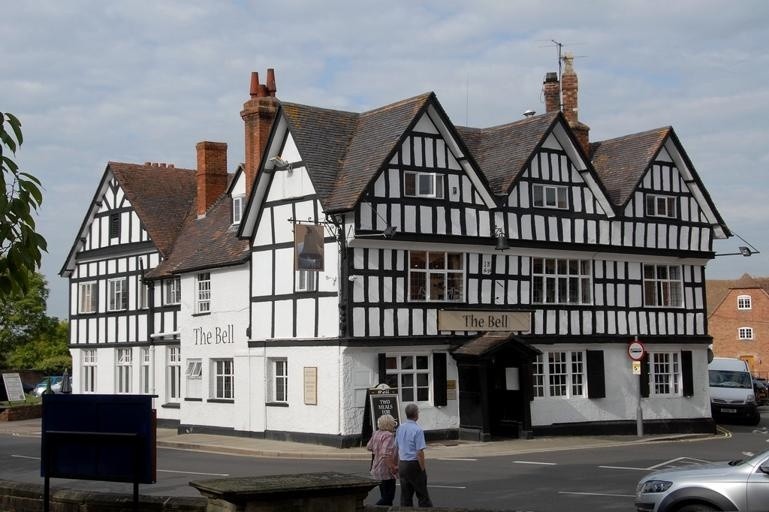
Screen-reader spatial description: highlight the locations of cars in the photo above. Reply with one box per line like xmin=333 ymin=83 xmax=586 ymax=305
xmin=634 ymin=449 xmax=769 ymax=512
xmin=23 ymin=376 xmax=72 ymax=397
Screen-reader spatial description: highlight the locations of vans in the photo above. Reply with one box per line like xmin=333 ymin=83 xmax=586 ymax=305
xmin=708 ymin=357 xmax=760 ymax=426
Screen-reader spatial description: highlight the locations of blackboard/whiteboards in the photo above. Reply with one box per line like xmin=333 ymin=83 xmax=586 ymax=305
xmin=370 ymin=394 xmax=401 ymax=437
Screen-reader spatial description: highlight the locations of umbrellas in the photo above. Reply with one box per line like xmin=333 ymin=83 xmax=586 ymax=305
xmin=59 ymin=367 xmax=73 ymax=394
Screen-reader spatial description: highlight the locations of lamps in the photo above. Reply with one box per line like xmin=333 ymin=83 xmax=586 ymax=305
xmin=354 ymin=225 xmax=397 ymax=239
xmin=270 ymin=156 xmax=293 ymax=174
xmin=716 ymin=248 xmax=752 ymax=256
xmin=495 ymin=226 xmax=510 ymax=250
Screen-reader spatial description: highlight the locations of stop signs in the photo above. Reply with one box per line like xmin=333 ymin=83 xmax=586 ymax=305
xmin=627 ymin=341 xmax=644 ymax=361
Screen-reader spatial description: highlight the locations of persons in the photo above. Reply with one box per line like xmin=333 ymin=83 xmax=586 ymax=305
xmin=391 ymin=403 xmax=432 ymax=507
xmin=365 ymin=413 xmax=399 ymax=505
